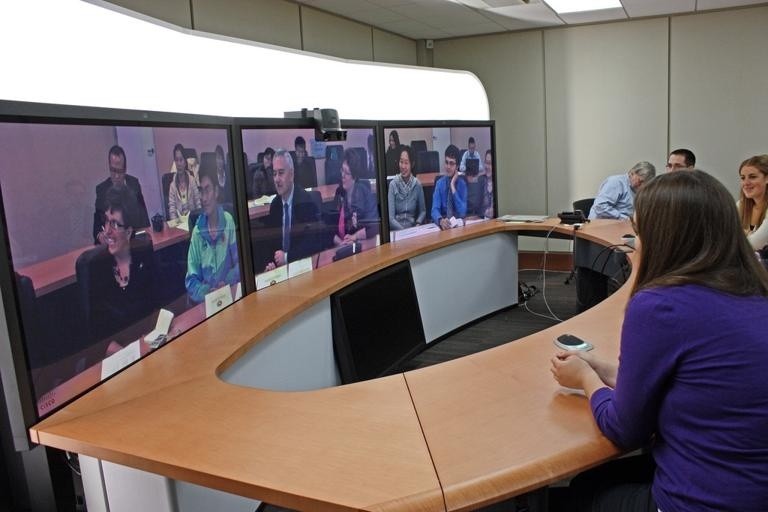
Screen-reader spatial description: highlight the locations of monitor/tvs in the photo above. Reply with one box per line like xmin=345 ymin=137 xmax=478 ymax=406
xmin=380 ymin=120 xmax=499 ymax=245
xmin=237 ymin=117 xmax=388 ymax=296
xmin=0 ymin=99 xmax=250 ymax=423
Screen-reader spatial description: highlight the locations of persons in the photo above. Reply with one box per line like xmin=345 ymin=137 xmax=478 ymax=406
xmin=327 ymin=147 xmax=379 ymax=244
xmin=385 ymin=130 xmax=494 ymax=231
xmin=475 ymin=150 xmax=493 ymax=218
xmin=735 ymin=154 xmax=768 ymax=269
xmin=587 ymin=161 xmax=656 ymax=220
xmin=460 ymin=136 xmax=483 ymax=170
xmin=255 ymin=149 xmax=326 ymax=270
xmin=88 ymin=187 xmax=180 ymax=332
xmin=168 ymin=142 xmax=230 ymax=220
xmin=432 ymin=145 xmax=467 ymax=228
xmin=184 ymin=162 xmax=241 ymax=306
xmin=245 ymin=138 xmax=318 ymax=199
xmin=385 ymin=131 xmax=427 ymax=230
xmin=93 ymin=145 xmax=150 ymax=243
xmin=551 ymin=170 xmax=768 ymax=512
xmin=667 ymin=149 xmax=696 ymax=173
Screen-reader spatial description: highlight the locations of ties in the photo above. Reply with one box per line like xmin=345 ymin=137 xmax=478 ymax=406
xmin=446 ymin=178 xmax=453 ymax=217
xmin=284 ymin=203 xmax=290 ymax=252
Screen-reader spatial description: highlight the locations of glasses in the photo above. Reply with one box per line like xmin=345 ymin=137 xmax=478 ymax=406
xmin=101 ymin=215 xmax=125 ymax=229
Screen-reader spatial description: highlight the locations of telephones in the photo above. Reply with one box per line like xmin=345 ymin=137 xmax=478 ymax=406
xmin=558 ymin=209 xmax=586 ymax=224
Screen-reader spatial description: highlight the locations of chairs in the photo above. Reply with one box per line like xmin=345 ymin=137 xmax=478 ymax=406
xmin=162 ymin=139 xmax=475 ymax=225
xmin=564 ymin=197 xmax=595 ymax=286
